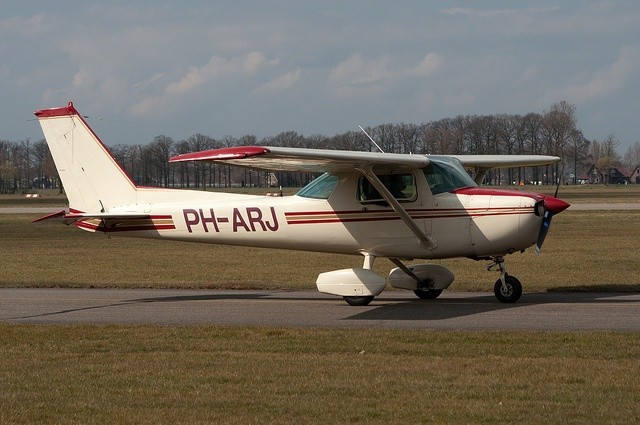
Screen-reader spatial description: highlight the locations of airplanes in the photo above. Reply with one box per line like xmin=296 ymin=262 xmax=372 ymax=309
xmin=34 ymin=101 xmax=571 ymax=307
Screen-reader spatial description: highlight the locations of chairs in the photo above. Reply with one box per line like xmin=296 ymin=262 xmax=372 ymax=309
xmin=362 ymin=180 xmax=401 ymax=205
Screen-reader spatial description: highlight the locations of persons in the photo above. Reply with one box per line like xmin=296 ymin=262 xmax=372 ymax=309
xmin=390 ymin=175 xmax=406 ymax=199
